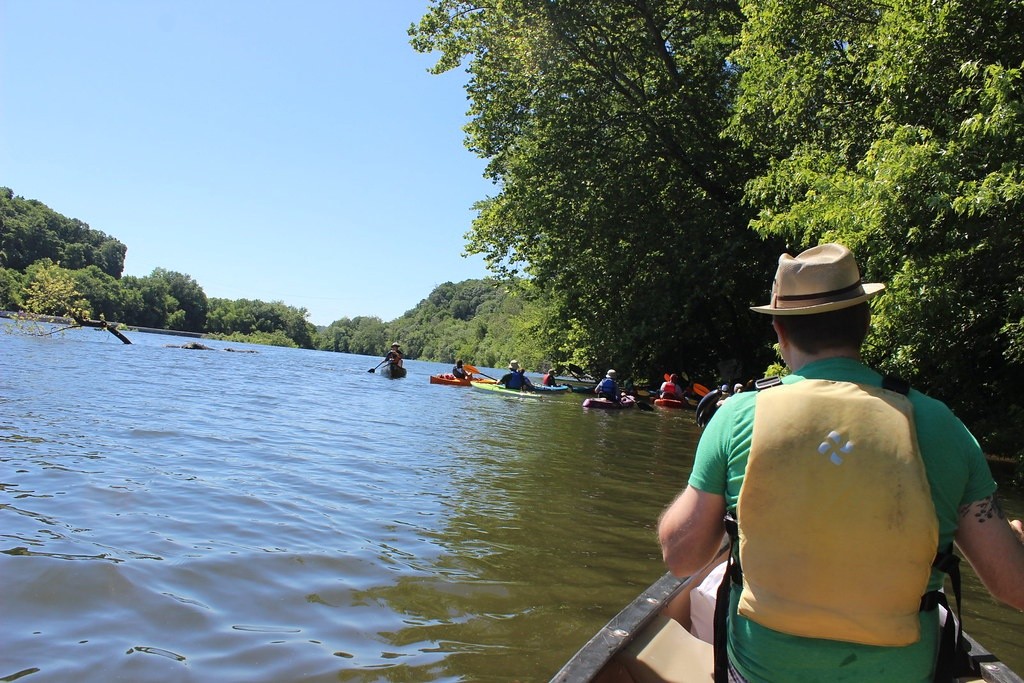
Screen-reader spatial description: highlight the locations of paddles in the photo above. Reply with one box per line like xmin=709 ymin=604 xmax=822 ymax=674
xmin=569 ymin=363 xmax=655 ymax=411
xmin=693 ymin=383 xmax=710 ymax=397
xmin=368 ymin=360 xmax=385 ymax=373
xmin=462 ymin=365 xmax=497 ymax=381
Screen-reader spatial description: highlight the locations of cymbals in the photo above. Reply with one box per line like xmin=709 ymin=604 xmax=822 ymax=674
xmin=663 ymin=374 xmax=671 ymax=382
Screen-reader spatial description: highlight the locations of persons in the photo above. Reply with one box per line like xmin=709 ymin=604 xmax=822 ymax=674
xmin=452 ymin=359 xmax=471 ymax=379
xmin=656 ymin=244 xmax=1024 ymax=683
xmin=594 ymin=368 xmax=757 ymax=408
xmin=382 ymin=342 xmax=402 ymax=368
xmin=496 ymin=360 xmax=529 ymax=390
xmin=543 ymin=369 xmax=555 ymax=386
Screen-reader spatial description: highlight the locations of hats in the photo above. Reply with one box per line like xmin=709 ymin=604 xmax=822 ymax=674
xmin=508 ymin=360 xmax=520 ymax=369
xmin=392 ymin=342 xmax=401 ymax=347
xmin=670 ymin=373 xmax=678 ymax=379
xmin=605 ymin=369 xmax=617 ymax=378
xmin=548 ymin=369 xmax=557 ymax=374
xmin=750 ymin=242 xmax=885 ymax=315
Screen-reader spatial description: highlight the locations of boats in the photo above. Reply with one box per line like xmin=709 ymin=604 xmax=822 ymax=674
xmin=548 ymin=529 xmax=1024 ymax=683
xmin=685 ymin=397 xmax=699 ymax=407
xmin=380 ymin=361 xmax=407 ymax=380
xmin=650 ymin=397 xmax=681 ymax=407
xmin=582 ymin=394 xmax=636 ymax=408
xmin=430 ymin=375 xmax=496 ymax=386
xmin=530 ymin=381 xmax=594 ymax=393
xmin=470 ymin=378 xmax=542 ymax=399
